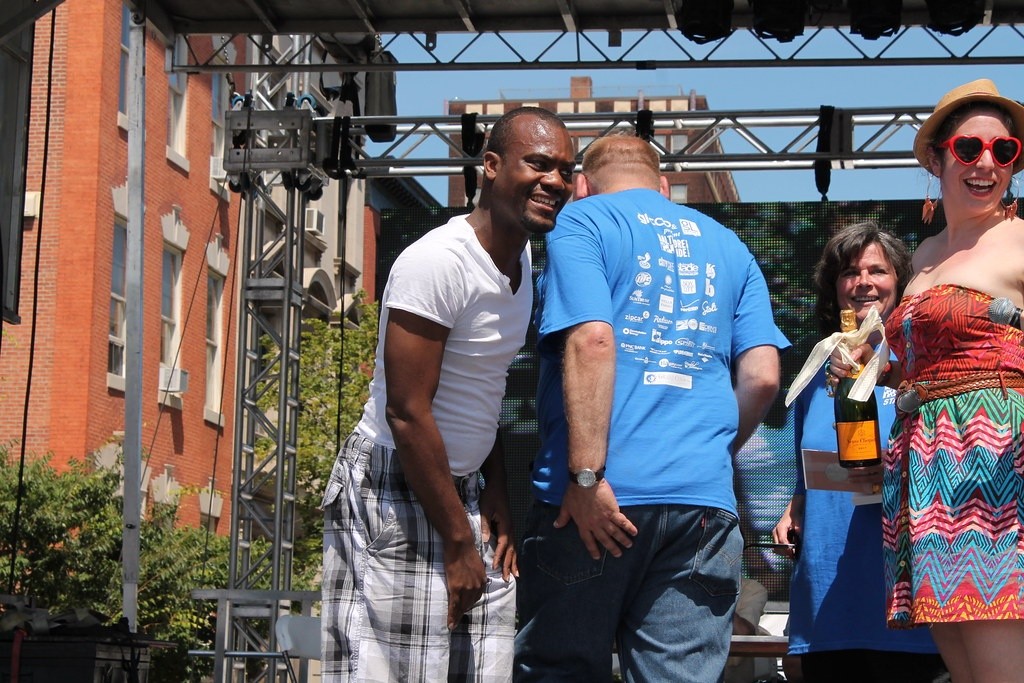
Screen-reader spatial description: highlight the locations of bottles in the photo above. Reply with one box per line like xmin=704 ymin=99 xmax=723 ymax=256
xmin=834 ymin=308 xmax=882 ymax=467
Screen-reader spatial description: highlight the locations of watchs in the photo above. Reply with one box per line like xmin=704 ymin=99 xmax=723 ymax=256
xmin=570 ymin=467 xmax=605 ymax=488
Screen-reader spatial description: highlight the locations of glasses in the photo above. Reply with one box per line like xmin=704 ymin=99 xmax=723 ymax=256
xmin=937 ymin=133 xmax=1022 ymax=168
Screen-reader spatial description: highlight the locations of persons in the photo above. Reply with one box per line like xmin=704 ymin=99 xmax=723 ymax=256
xmin=512 ymin=135 xmax=794 ymax=682
xmin=770 ymin=220 xmax=941 ymax=683
xmin=723 ymin=576 xmax=778 ymax=683
xmin=320 ymin=107 xmax=575 ymax=682
xmin=735 ymin=388 xmax=798 ymax=600
xmin=884 ymin=77 xmax=1024 ymax=683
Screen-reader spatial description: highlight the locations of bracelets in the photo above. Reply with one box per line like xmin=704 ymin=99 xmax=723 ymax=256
xmin=879 ymin=362 xmax=892 ymax=386
xmin=747 ymin=564 xmax=774 ymax=578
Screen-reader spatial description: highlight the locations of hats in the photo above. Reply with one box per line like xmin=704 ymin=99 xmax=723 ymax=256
xmin=913 ymin=79 xmax=1024 ymax=179
xmin=734 ymin=578 xmax=768 ymax=630
